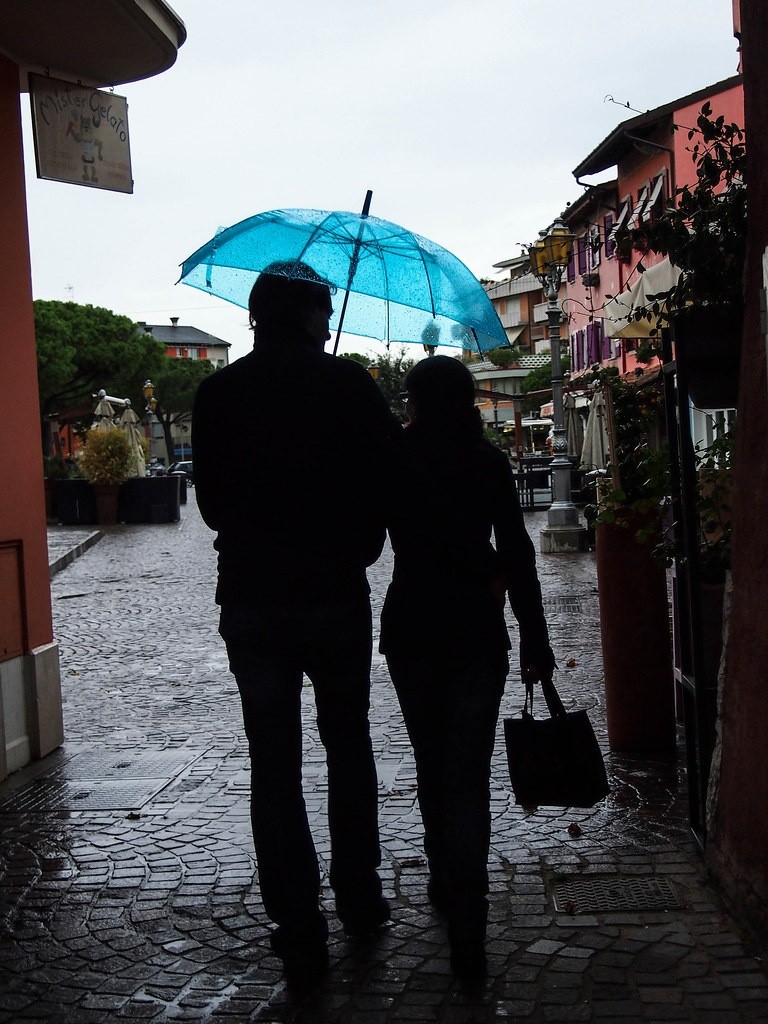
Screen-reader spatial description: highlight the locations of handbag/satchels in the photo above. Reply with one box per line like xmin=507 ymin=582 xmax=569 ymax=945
xmin=503 ymin=680 xmax=609 ymax=811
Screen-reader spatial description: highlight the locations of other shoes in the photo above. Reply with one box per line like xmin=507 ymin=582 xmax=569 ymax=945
xmin=343 ymin=901 xmax=389 ymax=935
xmin=269 ymin=933 xmax=328 ymax=973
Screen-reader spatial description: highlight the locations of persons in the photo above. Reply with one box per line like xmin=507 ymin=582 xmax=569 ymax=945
xmin=375 ymin=353 xmax=555 ymax=988
xmin=192 ymin=259 xmax=394 ymax=965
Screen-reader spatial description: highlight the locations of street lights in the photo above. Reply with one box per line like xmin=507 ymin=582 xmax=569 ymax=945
xmin=141 ymin=379 xmax=157 ymax=462
xmin=527 ymin=219 xmax=588 ymax=552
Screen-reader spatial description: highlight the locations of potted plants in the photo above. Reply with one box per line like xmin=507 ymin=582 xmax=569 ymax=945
xmin=72 ymin=423 xmax=138 ymax=525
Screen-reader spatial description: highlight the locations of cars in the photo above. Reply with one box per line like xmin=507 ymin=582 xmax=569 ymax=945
xmin=166 ymin=460 xmax=194 ymax=485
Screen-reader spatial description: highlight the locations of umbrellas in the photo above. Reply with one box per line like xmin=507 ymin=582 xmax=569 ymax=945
xmin=172 ymin=185 xmax=513 ymax=360
xmin=562 ymin=391 xmax=585 ymax=456
xmin=578 ymin=391 xmax=611 ymax=472
xmin=93 ymin=389 xmax=148 ymax=478
